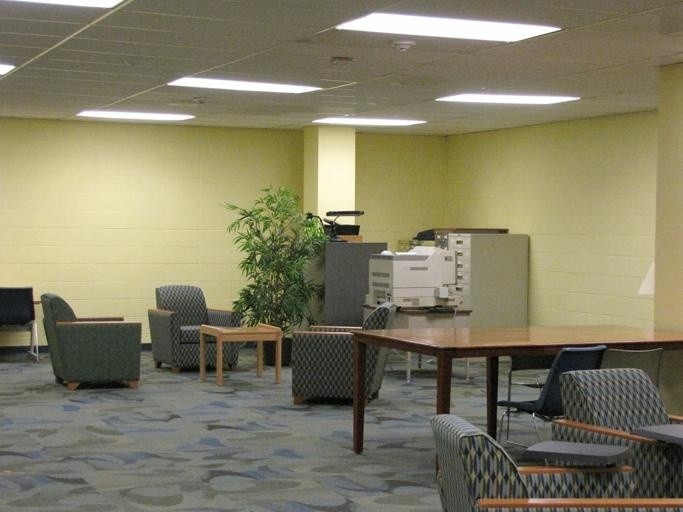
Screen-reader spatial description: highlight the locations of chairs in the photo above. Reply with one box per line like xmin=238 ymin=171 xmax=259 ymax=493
xmin=431 ymin=414 xmax=682 ymax=511
xmin=147 ymin=285 xmax=242 ymax=373
xmin=599 ymin=348 xmax=665 ymax=417
xmin=41 ymin=292 xmax=141 ymax=390
xmin=552 ymin=370 xmax=683 ymax=454
xmin=292 ymin=301 xmax=398 ymax=404
xmin=0 ymin=286 xmax=42 ymax=362
xmin=499 ymin=344 xmax=605 ymax=450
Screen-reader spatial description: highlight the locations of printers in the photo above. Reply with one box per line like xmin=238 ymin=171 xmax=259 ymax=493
xmin=368 ymin=246 xmax=457 ymax=308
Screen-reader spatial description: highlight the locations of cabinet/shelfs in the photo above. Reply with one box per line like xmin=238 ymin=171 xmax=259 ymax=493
xmin=448 ymin=232 xmax=529 ymax=363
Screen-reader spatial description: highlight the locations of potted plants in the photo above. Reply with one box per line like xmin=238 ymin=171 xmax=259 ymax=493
xmin=217 ymin=182 xmax=331 ymax=366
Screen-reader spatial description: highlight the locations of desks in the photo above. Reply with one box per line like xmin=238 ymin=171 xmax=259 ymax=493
xmin=363 ymin=304 xmax=473 ymax=383
xmin=350 ymin=324 xmax=682 ymax=453
xmin=198 ymin=322 xmax=284 ymax=386
